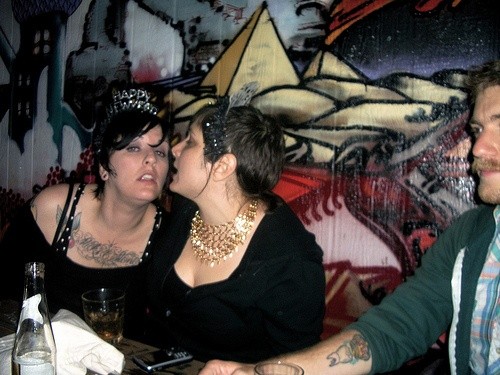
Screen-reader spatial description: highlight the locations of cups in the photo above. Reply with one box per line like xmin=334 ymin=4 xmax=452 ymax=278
xmin=81 ymin=288 xmax=127 ymax=344
xmin=254 ymin=361 xmax=304 ymax=375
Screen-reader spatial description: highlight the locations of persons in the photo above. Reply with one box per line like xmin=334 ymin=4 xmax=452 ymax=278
xmin=0 ymin=81 xmax=203 ymax=339
xmin=140 ymin=105 xmax=326 ymax=363
xmin=198 ymin=60 xmax=500 ymax=375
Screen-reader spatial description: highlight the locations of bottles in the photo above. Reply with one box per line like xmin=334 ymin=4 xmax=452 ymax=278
xmin=11 ymin=262 xmax=57 ymax=375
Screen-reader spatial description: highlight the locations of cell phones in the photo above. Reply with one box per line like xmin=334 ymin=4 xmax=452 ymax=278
xmin=131 ymin=343 xmax=193 ymax=373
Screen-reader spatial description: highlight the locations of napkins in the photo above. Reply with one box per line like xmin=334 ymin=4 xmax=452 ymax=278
xmin=51 ymin=309 xmax=127 ymax=375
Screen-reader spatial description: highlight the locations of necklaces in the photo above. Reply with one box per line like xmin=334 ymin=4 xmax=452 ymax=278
xmin=191 ymin=199 xmax=258 ymax=265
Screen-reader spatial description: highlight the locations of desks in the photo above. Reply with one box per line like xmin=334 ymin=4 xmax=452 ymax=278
xmin=0 ymin=321 xmax=206 ymax=375
